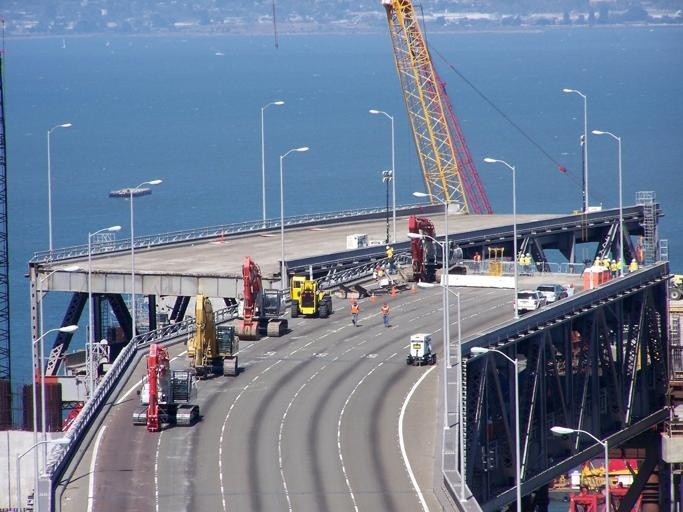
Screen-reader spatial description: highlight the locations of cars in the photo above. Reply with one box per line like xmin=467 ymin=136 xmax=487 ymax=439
xmin=536 ymin=284 xmax=568 ymax=303
xmin=513 ymin=291 xmax=547 ymax=315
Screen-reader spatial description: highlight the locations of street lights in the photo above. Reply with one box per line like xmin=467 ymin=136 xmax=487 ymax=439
xmin=279 ymin=147 xmax=310 ymax=298
xmin=129 ymin=179 xmax=163 ymax=339
xmin=471 ymin=346 xmax=521 ymax=512
xmin=417 ymin=282 xmax=464 ymax=501
xmin=40 ymin=266 xmax=80 ymax=472
xmin=89 ymin=225 xmax=123 ymax=396
xmin=550 ymin=427 xmax=610 ymax=511
xmin=369 ymin=109 xmax=396 ymax=244
xmin=407 ymin=232 xmax=450 ymax=430
xmin=563 ymin=88 xmax=587 ymax=214
xmin=261 ymin=101 xmax=284 ymax=228
xmin=15 ymin=439 xmax=71 ymax=512
xmin=32 ymin=325 xmax=78 ymax=512
xmin=413 ymin=191 xmax=451 ymax=369
xmin=382 ymin=170 xmax=394 ymax=242
xmin=483 ymin=157 xmax=519 ymax=318
xmin=592 ymin=130 xmax=624 ymax=275
xmin=47 ymin=122 xmax=72 ymax=256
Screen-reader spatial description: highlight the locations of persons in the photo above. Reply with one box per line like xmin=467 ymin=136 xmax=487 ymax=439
xmin=381 ymin=301 xmax=391 ymax=327
xmin=385 ymin=246 xmax=394 ymax=264
xmin=525 ymin=253 xmax=533 ymax=276
xmin=593 ymin=255 xmax=624 ymax=279
xmin=370 ymin=293 xmax=378 ymax=308
xmin=629 ymin=259 xmax=639 ymax=273
xmin=519 ymin=254 xmax=526 ymax=276
xmin=473 ymin=251 xmax=481 ymax=273
xmin=372 ymin=264 xmax=397 ymax=281
xmin=351 ymin=301 xmax=359 ymax=327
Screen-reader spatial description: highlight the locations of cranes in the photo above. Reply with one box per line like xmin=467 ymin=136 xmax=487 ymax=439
xmin=383 ymin=1 xmax=493 ymax=215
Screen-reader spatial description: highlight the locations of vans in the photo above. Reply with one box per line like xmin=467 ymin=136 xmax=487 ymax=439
xmin=406 ymin=334 xmax=436 ymax=366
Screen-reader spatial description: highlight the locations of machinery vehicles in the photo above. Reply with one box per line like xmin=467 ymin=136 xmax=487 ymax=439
xmin=185 ymin=295 xmax=237 ymax=377
xmin=290 ymin=274 xmax=332 ymax=317
xmin=668 ymin=275 xmax=683 ymax=300
xmin=406 ymin=216 xmax=467 ymax=282
xmin=132 ymin=343 xmax=199 ymax=431
xmin=234 ymin=257 xmax=288 ymax=340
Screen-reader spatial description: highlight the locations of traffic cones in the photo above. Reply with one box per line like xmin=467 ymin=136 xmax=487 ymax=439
xmin=351 ymin=294 xmax=356 ymax=304
xmin=411 ymin=286 xmax=416 ymax=293
xmin=371 ymin=293 xmax=377 ymax=303
xmin=392 ymin=287 xmax=397 ymax=296
